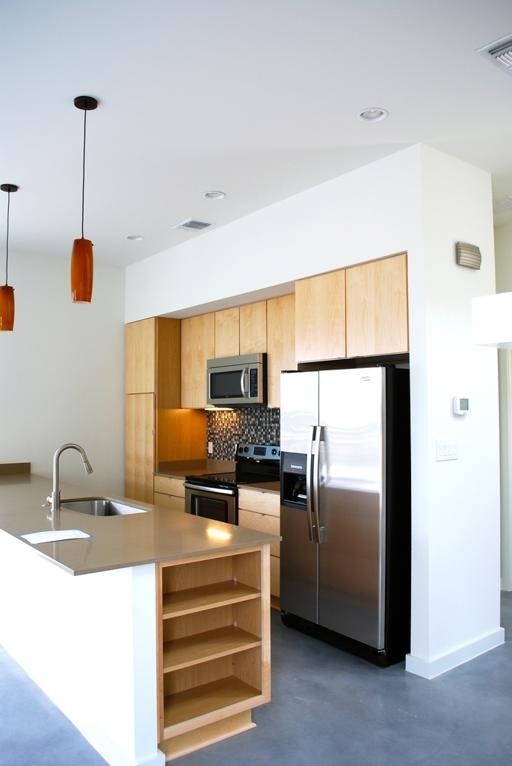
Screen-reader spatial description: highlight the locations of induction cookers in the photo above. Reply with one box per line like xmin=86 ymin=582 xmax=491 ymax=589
xmin=185 ymin=444 xmax=280 ymax=484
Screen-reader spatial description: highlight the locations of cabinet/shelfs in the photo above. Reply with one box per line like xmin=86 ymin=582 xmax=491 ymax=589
xmin=266 ymin=295 xmax=296 ymax=408
xmin=214 ymin=299 xmax=266 ymax=358
xmin=237 ymin=489 xmax=280 ymax=598
xmin=126 ymin=316 xmax=180 ymax=504
xmin=294 ymin=254 xmax=408 ymax=363
xmin=155 ymin=544 xmax=273 ymax=763
xmin=154 ymin=476 xmax=186 ymax=513
xmin=180 ymin=313 xmax=215 ymax=409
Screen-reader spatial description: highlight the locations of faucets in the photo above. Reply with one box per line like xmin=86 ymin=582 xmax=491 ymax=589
xmin=43 ymin=441 xmax=95 ymax=512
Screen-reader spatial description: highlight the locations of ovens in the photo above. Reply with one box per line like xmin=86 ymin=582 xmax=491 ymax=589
xmin=184 ymin=482 xmax=238 ymax=525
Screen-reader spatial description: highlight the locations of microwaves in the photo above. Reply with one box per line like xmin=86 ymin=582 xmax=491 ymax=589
xmin=206 ymin=353 xmax=265 ymax=408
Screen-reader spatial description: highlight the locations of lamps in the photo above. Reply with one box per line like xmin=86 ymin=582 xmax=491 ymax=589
xmin=71 ymin=95 xmax=99 ymax=304
xmin=0 ymin=183 xmax=20 ymax=333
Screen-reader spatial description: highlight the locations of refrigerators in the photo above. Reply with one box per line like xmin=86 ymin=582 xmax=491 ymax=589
xmin=279 ymin=364 xmax=411 ymax=668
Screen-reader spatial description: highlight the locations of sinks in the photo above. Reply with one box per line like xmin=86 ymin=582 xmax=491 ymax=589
xmin=59 ymin=494 xmax=154 ymax=517
